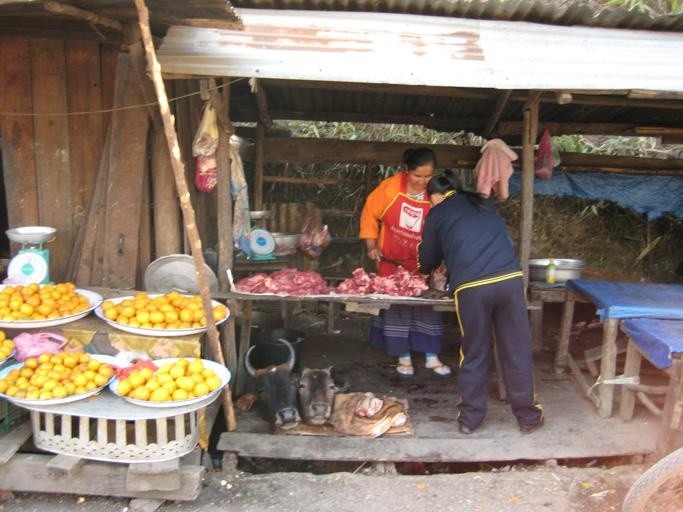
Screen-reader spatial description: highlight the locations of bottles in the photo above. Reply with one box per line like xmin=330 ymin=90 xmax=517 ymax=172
xmin=545 ymin=258 xmax=556 ymax=284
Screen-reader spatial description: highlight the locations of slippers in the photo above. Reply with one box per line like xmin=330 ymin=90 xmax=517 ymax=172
xmin=395 ymin=361 xmax=415 ymax=378
xmin=428 ymin=362 xmax=453 ymax=378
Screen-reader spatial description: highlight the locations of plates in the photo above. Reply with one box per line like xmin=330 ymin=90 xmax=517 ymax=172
xmin=0 ymin=343 xmax=17 ymax=366
xmin=109 ymin=358 xmax=232 ymax=409
xmin=0 ymin=282 xmax=104 ymax=328
xmin=0 ymin=355 xmax=121 ymax=406
xmin=96 ymin=295 xmax=231 ymax=336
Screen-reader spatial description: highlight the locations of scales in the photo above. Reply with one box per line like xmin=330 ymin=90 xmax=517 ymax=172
xmin=3 ymin=225 xmax=58 ymax=287
xmin=240 ymin=209 xmax=278 ymax=259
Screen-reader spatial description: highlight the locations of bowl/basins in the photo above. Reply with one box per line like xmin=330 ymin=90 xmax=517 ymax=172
xmin=528 ymin=259 xmax=585 ymax=284
xmin=272 ymin=232 xmax=300 ymax=250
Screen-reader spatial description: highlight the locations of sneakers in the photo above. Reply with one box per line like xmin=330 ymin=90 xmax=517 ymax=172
xmin=458 ymin=422 xmax=471 ymax=434
xmin=520 ymin=404 xmax=544 ymax=433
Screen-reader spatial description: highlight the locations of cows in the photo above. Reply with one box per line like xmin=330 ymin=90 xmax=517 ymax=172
xmin=298 ymin=366 xmax=349 ymax=426
xmin=245 ymin=337 xmax=300 ymax=430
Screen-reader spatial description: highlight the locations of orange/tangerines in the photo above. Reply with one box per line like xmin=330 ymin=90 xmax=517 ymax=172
xmin=0 ymin=330 xmax=16 ymax=365
xmin=100 ymin=290 xmax=228 ymax=331
xmin=0 ymin=280 xmax=93 ymax=324
xmin=0 ymin=353 xmax=116 ymax=403
xmin=115 ymin=355 xmax=221 ymax=403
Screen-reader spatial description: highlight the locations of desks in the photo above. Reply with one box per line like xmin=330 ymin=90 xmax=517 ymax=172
xmin=211 ymin=287 xmax=507 ymax=402
xmin=552 ymin=280 xmax=683 ymax=416
xmin=619 ymin=319 xmax=683 ymax=428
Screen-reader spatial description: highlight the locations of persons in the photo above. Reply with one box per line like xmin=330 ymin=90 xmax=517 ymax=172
xmin=417 ymin=170 xmax=546 ymax=432
xmin=360 ymin=149 xmax=453 ymax=375
